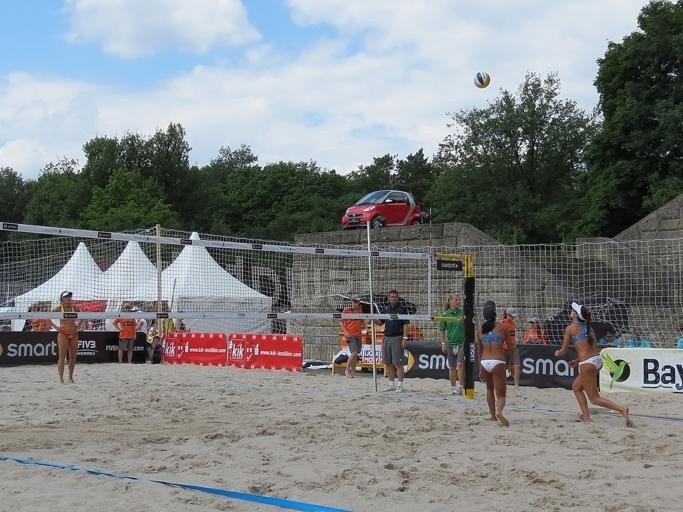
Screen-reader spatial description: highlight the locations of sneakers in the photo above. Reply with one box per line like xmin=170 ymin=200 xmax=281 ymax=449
xmin=453 ymin=385 xmax=463 ymax=394
xmin=384 ymin=386 xmax=395 ymax=391
xmin=396 ymin=386 xmax=403 ymax=392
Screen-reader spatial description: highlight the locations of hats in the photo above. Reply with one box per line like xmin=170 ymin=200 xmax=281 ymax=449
xmin=506 ymin=307 xmax=519 ymax=317
xmin=352 ymin=294 xmax=360 ymax=300
xmin=530 ymin=317 xmax=539 ymax=323
xmin=571 ymin=301 xmax=586 ymax=321
xmin=63 ymin=291 xmax=73 ymax=297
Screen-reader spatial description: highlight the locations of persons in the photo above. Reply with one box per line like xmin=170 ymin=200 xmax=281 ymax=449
xmin=478 ymin=301 xmax=515 ymax=426
xmin=440 ymin=292 xmax=465 ymax=394
xmin=676 ymin=321 xmax=683 ymax=350
xmin=337 ymin=295 xmax=366 ymax=378
xmin=131 ymin=306 xmax=166 ymax=363
xmin=642 ymin=328 xmax=656 ymax=349
xmin=625 ymin=326 xmax=651 ymax=348
xmin=523 ymin=316 xmax=545 ymax=343
xmin=111 ymin=302 xmax=138 ymax=363
xmin=598 ymin=324 xmax=620 ymax=347
xmin=49 ymin=291 xmax=80 ymax=385
xmin=555 ymin=302 xmax=630 ymax=426
xmin=500 ymin=306 xmax=518 ymax=344
xmin=372 ymin=290 xmax=410 ymax=392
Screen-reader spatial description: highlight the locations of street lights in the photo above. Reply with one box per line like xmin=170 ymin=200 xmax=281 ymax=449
xmin=418 ymin=198 xmax=432 ymax=324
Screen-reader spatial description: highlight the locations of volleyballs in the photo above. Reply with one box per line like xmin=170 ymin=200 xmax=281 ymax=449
xmin=474 ymin=72 xmax=490 ymax=88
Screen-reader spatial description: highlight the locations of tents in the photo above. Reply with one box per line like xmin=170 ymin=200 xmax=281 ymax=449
xmin=70 ymin=241 xmax=160 ymax=330
xmin=13 ymin=240 xmax=105 ymax=332
xmin=117 ymin=231 xmax=274 ymax=334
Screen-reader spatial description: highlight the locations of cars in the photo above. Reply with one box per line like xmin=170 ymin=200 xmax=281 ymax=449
xmin=341 ymin=190 xmax=422 ymax=229
xmin=0 ymin=299 xmax=15 ymax=312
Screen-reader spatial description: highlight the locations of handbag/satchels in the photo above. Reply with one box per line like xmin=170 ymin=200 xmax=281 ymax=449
xmin=335 ymin=354 xmax=349 ymax=364
